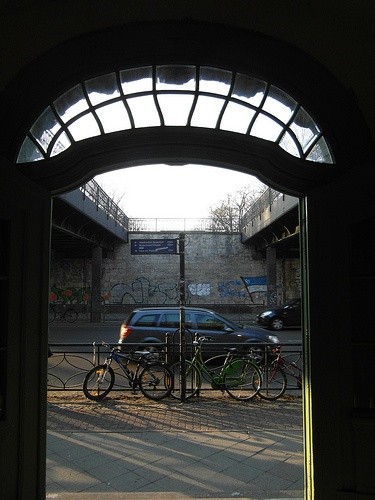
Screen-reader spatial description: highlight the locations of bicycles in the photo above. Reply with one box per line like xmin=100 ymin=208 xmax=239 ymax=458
xmin=48 ymin=307 xmax=79 ymax=323
xmin=82 ymin=335 xmax=303 ymax=402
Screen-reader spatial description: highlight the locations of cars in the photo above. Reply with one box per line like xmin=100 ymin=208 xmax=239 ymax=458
xmin=256 ymin=297 xmax=301 ymax=332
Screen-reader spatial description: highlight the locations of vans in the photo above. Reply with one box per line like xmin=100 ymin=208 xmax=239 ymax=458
xmin=117 ymin=307 xmax=282 ymax=366
